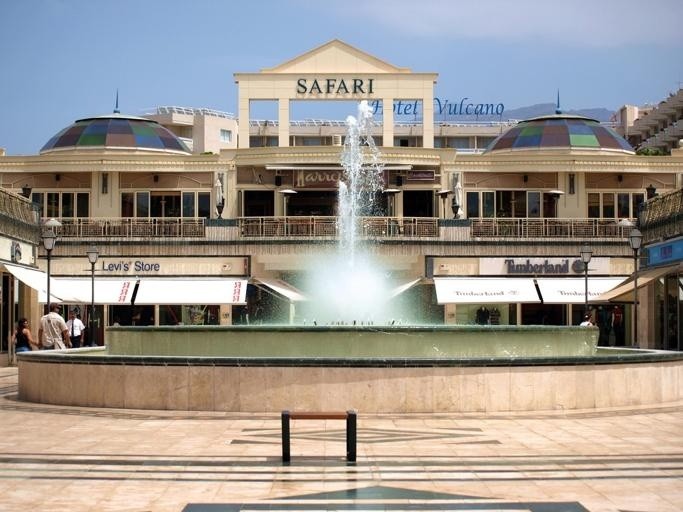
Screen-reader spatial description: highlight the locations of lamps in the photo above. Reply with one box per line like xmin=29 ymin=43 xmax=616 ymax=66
xmin=383 ymin=189 xmax=401 ymax=216
xmin=615 ymin=218 xmax=640 ymax=229
xmin=278 ymin=190 xmax=298 ymax=216
xmin=551 ymin=190 xmax=564 ymax=202
xmin=435 ymin=190 xmax=455 ymax=220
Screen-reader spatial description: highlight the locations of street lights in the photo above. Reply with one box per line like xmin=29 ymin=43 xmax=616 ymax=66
xmin=86 ymin=244 xmax=99 ymax=347
xmin=579 ymin=243 xmax=594 ymax=312
xmin=40 ymin=230 xmax=58 ymax=313
xmin=627 ymin=228 xmax=643 ymax=347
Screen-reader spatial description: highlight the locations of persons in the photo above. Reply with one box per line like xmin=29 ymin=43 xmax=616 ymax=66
xmin=580 ymin=312 xmax=593 ymax=326
xmin=65 ymin=311 xmax=85 ymax=348
xmin=588 ymin=305 xmax=622 ymax=328
xmin=37 ymin=303 xmax=70 ymax=350
xmin=477 ymin=306 xmax=489 ymax=325
xmin=489 ymin=307 xmax=501 ymax=325
xmin=73 ymin=305 xmax=81 ymax=319
xmin=86 ymin=307 xmax=100 ymax=347
xmin=12 ymin=318 xmax=33 ymax=353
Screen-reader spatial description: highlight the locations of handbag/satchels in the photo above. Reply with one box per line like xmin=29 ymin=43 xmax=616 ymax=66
xmin=55 ymin=340 xmax=66 ymax=349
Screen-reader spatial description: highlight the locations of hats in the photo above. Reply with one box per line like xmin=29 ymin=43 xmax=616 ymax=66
xmin=50 ymin=303 xmax=61 ymax=309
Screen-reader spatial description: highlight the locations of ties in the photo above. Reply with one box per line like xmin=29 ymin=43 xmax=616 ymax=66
xmin=72 ymin=321 xmax=75 ymax=336
